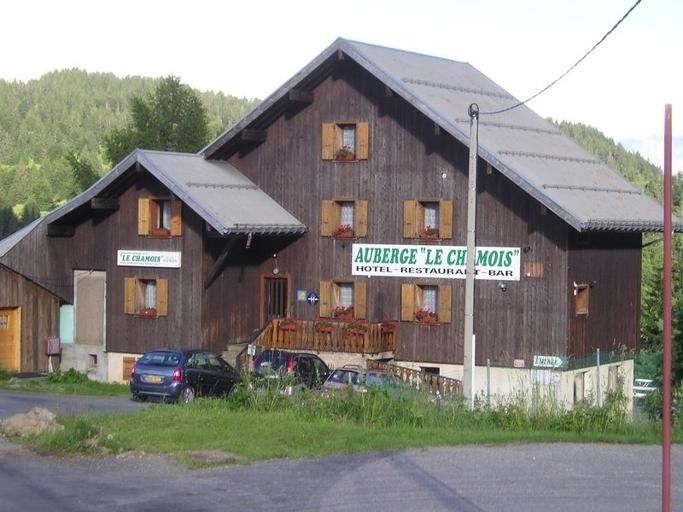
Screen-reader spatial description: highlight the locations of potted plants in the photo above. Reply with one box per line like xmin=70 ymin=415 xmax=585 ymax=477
xmin=140 ymin=306 xmax=156 ymax=316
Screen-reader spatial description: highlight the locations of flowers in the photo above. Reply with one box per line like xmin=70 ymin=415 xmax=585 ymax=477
xmin=334 ymin=305 xmax=354 ymax=315
xmin=280 ymin=316 xmax=295 ymax=324
xmin=414 ymin=307 xmax=435 ymax=318
xmin=313 ymin=315 xmax=334 ymax=328
xmin=381 ymin=318 xmax=400 ymax=328
xmin=422 ymin=225 xmax=439 ymax=235
xmin=347 ymin=321 xmax=367 ymax=330
xmin=334 ymin=224 xmax=353 ymax=234
xmin=339 ymin=142 xmax=352 ymax=157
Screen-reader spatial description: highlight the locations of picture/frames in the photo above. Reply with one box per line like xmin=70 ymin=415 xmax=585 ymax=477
xmin=336 ymin=153 xmax=354 ymax=160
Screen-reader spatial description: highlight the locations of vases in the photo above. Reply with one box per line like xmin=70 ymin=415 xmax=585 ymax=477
xmin=426 ymin=233 xmax=438 ymax=240
xmin=316 ymin=326 xmax=332 ymax=333
xmin=337 ymin=232 xmax=353 ymax=237
xmin=339 ymin=314 xmax=353 ymax=320
xmin=422 ymin=316 xmax=436 ymax=322
xmin=384 ymin=326 xmax=393 ymax=333
xmin=282 ymin=325 xmax=295 ymax=330
xmin=351 ymin=329 xmax=364 ymax=334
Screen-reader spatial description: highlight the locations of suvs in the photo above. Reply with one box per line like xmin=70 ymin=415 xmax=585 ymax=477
xmin=129 ymin=348 xmax=243 ymax=406
xmin=321 ymin=368 xmax=417 ymax=403
xmin=248 ymin=350 xmax=332 ymax=397
